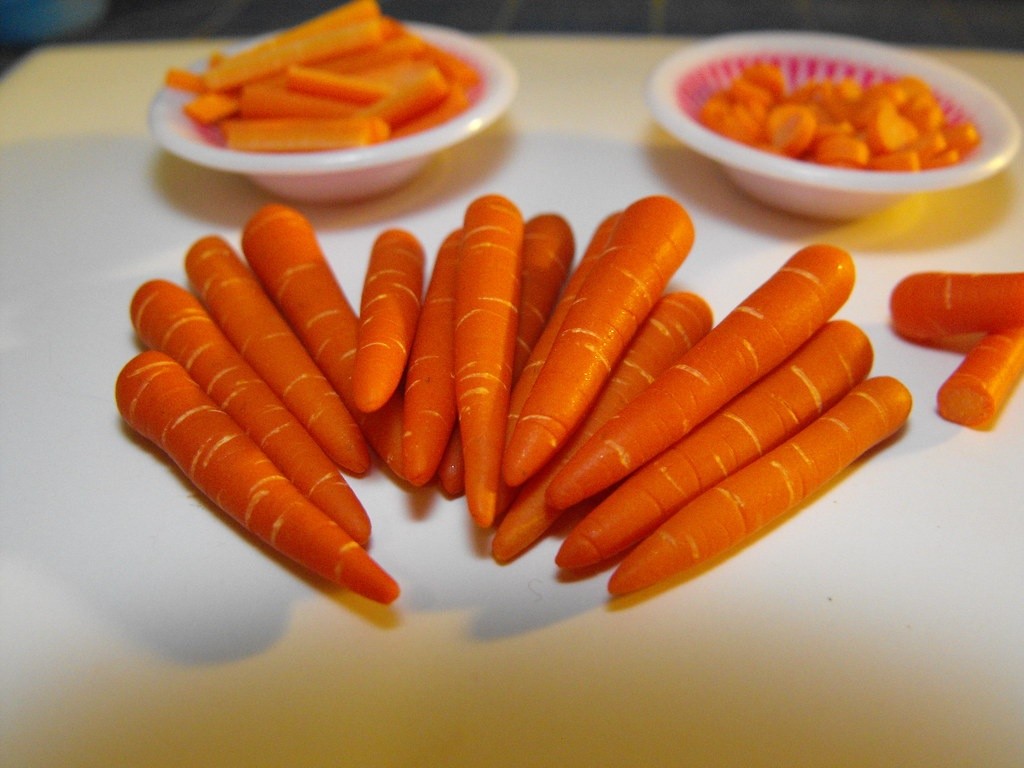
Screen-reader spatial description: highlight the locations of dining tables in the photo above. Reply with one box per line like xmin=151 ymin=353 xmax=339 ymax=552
xmin=1 ymin=37 xmax=1024 ymax=768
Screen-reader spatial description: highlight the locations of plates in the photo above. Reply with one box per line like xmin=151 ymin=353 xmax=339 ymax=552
xmin=148 ymin=17 xmax=520 ymax=206
xmin=643 ymin=30 xmax=1023 ymax=224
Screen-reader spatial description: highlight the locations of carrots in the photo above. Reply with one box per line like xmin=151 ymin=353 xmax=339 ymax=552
xmin=115 ymin=0 xmax=1024 ymax=607
